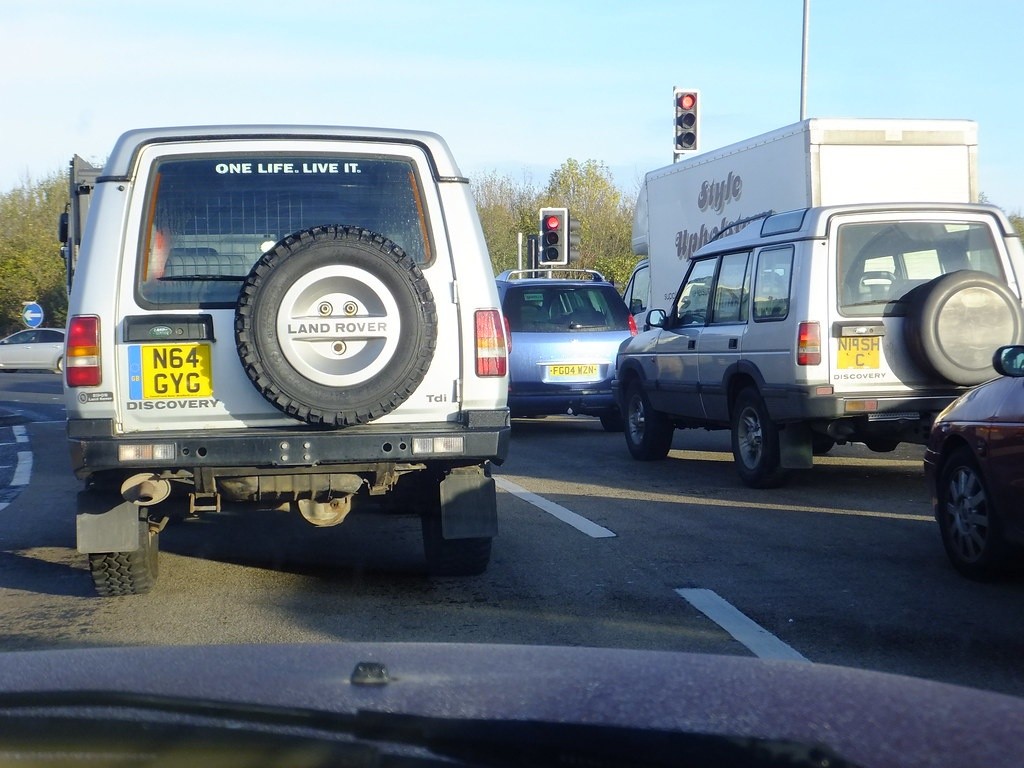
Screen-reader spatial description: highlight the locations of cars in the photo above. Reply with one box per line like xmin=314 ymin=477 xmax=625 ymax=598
xmin=924 ymin=345 xmax=1024 ymax=577
xmin=0 ymin=328 xmax=65 ymax=374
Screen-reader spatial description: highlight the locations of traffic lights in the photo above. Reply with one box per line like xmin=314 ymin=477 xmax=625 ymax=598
xmin=676 ymin=92 xmax=697 ymax=150
xmin=544 ymin=215 xmax=563 ymax=262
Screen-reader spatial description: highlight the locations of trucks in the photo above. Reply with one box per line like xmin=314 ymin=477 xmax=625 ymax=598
xmin=623 ymin=119 xmax=978 ymax=334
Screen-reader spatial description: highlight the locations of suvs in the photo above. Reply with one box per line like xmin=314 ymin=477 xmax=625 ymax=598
xmin=62 ymin=124 xmax=512 ymax=598
xmin=611 ymin=202 xmax=1024 ymax=489
xmin=494 ymin=268 xmax=639 ymax=431
